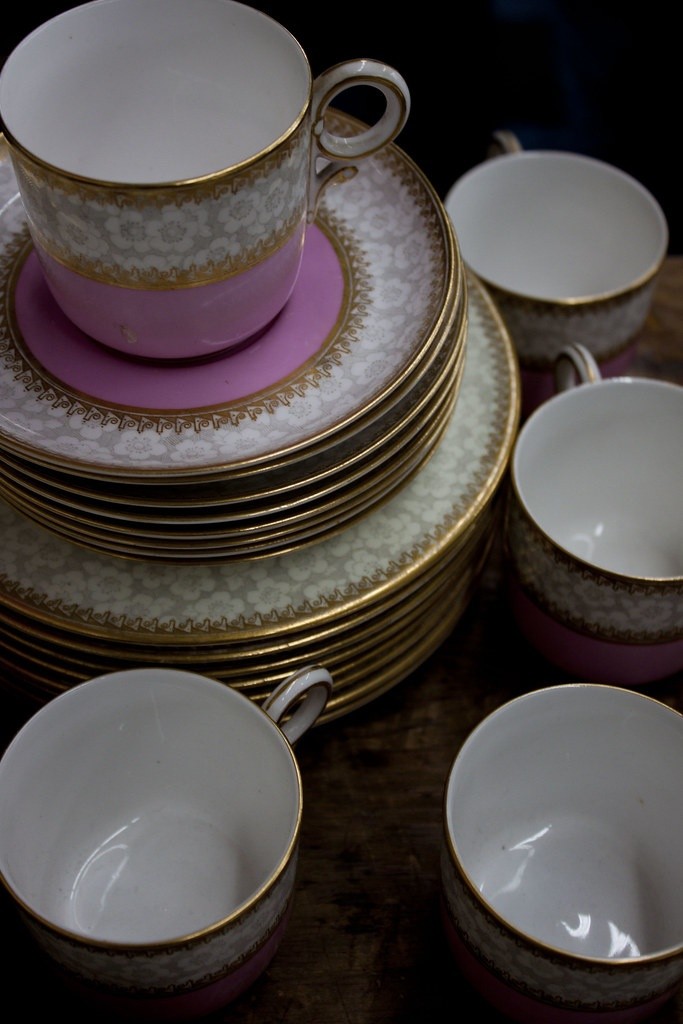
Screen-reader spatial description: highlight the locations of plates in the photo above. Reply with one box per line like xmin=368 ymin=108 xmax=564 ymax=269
xmin=0 ymin=111 xmax=521 ymax=733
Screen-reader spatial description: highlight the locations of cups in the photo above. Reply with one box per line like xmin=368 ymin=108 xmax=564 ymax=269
xmin=0 ymin=0 xmax=412 ymax=364
xmin=1 ymin=662 xmax=333 ymax=1017
xmin=440 ymin=688 xmax=683 ymax=1024
xmin=512 ymin=343 xmax=683 ymax=686
xmin=444 ymin=124 xmax=669 ymax=408
